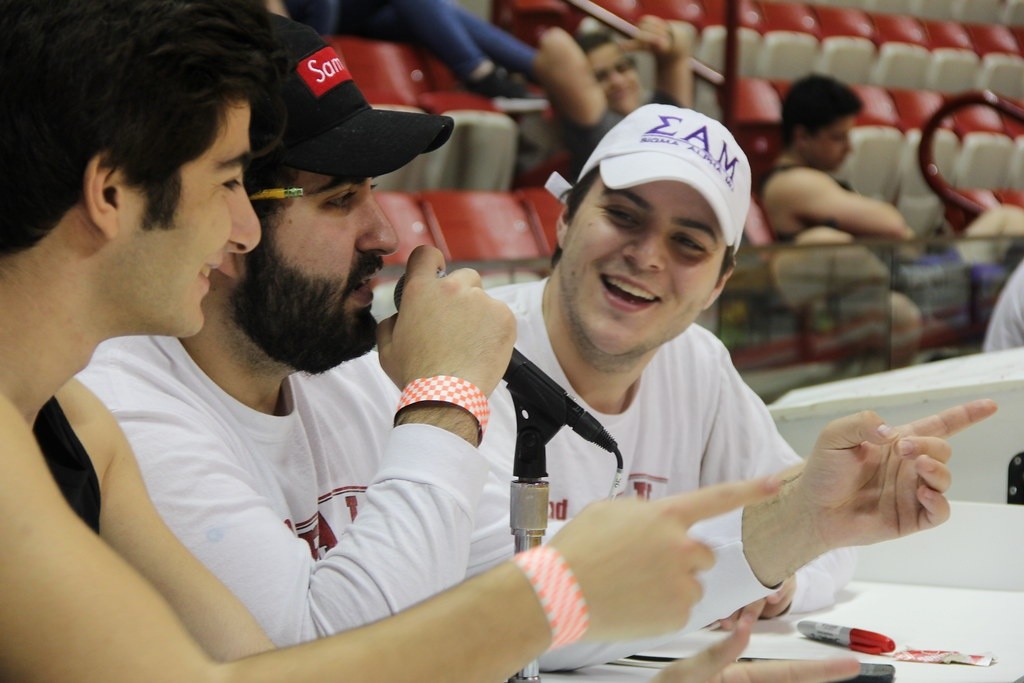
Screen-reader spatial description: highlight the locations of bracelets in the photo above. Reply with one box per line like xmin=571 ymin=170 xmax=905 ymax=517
xmin=513 ymin=546 xmax=589 ymax=652
xmin=394 ymin=376 xmax=490 ymax=447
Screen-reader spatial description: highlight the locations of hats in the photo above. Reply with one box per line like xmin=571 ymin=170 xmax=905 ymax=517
xmin=266 ymin=12 xmax=454 ymax=178
xmin=544 ymin=102 xmax=751 ymax=256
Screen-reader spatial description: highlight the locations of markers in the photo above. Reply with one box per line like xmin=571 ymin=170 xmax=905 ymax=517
xmin=796 ymin=620 xmax=896 ymax=656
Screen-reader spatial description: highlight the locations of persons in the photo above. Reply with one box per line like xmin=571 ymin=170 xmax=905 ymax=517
xmin=1 ymin=0 xmax=1024 ymax=683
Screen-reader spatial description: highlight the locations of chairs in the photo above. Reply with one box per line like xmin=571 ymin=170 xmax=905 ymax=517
xmin=332 ymin=0 xmax=1024 ymax=395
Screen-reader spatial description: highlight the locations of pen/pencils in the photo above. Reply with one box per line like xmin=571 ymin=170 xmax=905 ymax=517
xmin=248 ymin=186 xmax=304 ymax=200
xmin=738 ymin=657 xmax=896 ymax=683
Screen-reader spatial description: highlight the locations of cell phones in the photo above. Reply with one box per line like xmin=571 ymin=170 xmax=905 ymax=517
xmin=740 ymin=657 xmax=896 ymax=683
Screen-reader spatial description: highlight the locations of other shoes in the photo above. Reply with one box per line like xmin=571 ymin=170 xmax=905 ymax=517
xmin=466 ymin=65 xmax=530 ymax=99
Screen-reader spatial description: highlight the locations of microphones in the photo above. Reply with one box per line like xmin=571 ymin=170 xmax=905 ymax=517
xmin=394 ymin=267 xmax=619 ymax=454
